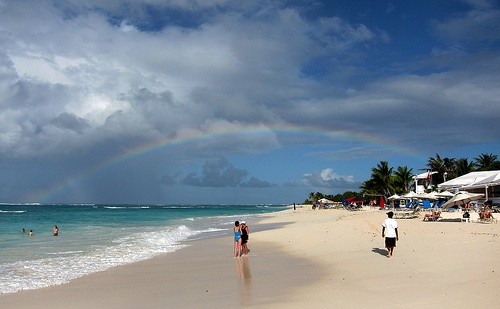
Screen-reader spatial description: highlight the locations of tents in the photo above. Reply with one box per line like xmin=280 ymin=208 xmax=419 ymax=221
xmin=438 ymin=170 xmax=500 ymax=203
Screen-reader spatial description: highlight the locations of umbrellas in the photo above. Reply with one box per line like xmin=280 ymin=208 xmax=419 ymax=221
xmin=388 ymin=190 xmax=455 ymax=200
xmin=318 ymin=198 xmax=327 ymax=203
xmin=442 ymin=192 xmax=485 ymax=213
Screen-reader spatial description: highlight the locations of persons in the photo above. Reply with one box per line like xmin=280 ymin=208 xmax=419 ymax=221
xmin=312 ymin=199 xmax=377 ymax=210
xmin=22 ymin=225 xmax=60 ymax=237
xmin=422 ymin=206 xmax=493 ymax=222
xmin=381 ymin=212 xmax=399 ymax=257
xmin=233 ymin=221 xmax=250 ymax=259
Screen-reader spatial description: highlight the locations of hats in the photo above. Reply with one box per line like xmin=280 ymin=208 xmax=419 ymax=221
xmin=386 ymin=212 xmax=394 ymax=215
xmin=240 ymin=221 xmax=245 ymax=225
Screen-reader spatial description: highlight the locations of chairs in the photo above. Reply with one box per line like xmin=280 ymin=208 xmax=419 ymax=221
xmin=393 ymin=203 xmax=498 ymax=225
